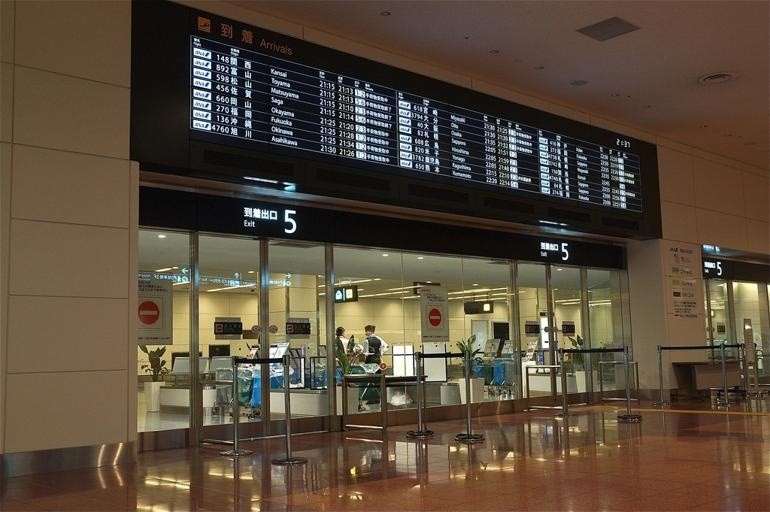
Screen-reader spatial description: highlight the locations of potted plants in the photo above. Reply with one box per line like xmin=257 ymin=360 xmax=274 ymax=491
xmin=455 ymin=333 xmax=486 ymax=403
xmin=568 ymin=334 xmax=598 ymax=392
xmin=334 ymin=335 xmax=360 ymax=415
xmin=138 ymin=344 xmax=172 ymax=413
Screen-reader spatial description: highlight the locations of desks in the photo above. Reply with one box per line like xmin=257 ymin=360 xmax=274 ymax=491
xmin=342 ymin=373 xmax=428 ymax=417
xmin=671 ymin=358 xmax=742 ymax=404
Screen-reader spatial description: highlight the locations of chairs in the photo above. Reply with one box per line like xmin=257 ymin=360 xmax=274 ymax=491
xmin=169 ymin=356 xmax=236 ymax=390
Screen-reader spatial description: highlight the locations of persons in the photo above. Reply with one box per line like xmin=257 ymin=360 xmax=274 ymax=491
xmin=362 ymin=325 xmax=390 ymax=373
xmin=335 ymin=326 xmax=364 ymax=357
